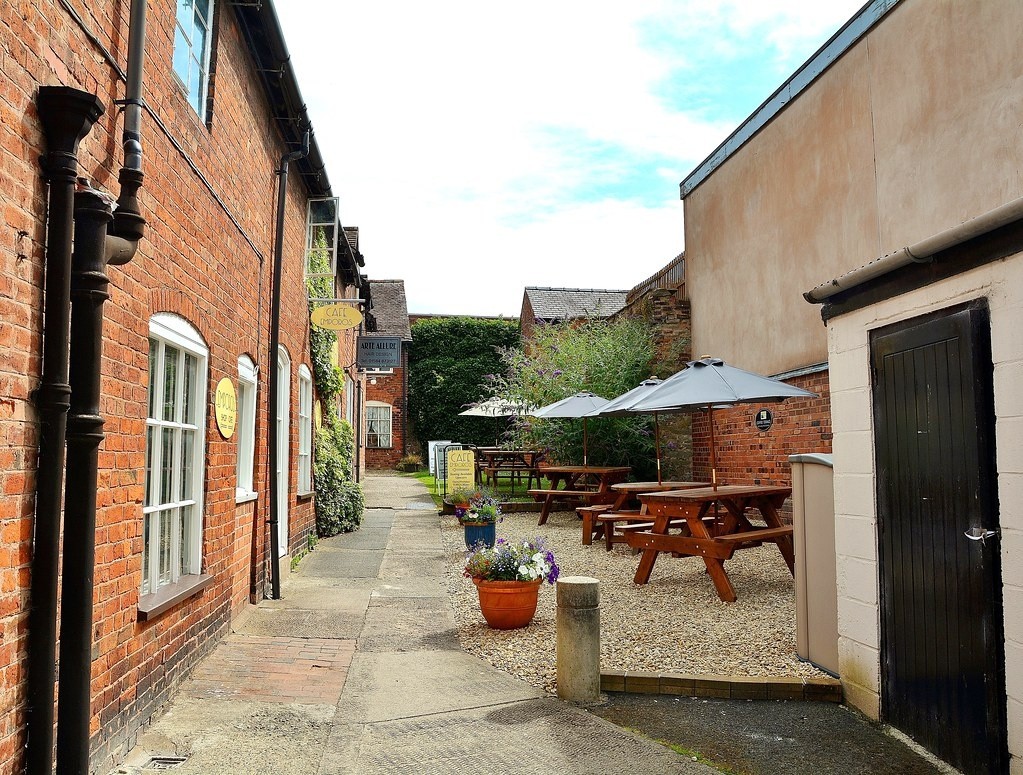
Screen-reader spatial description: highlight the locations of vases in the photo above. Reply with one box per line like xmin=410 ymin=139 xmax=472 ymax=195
xmin=463 ymin=521 xmax=497 ymax=551
xmin=471 ymin=577 xmax=543 ymax=629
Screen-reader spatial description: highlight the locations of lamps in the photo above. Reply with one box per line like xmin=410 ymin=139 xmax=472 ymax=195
xmin=357 ymin=377 xmax=377 ymax=386
xmin=342 ymin=362 xmax=366 ymax=379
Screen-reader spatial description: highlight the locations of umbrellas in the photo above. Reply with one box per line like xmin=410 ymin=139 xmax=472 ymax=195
xmin=627 ymin=353 xmax=820 ymax=540
xmin=525 ymin=388 xmax=612 ymax=491
xmin=582 ymin=376 xmax=735 ymax=485
xmin=456 ymin=396 xmax=543 ymax=460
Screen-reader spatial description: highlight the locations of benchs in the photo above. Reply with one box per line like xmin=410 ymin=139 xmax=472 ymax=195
xmin=474 ymin=461 xmax=525 ymax=464
xmin=598 ymin=513 xmax=673 ymax=521
xmin=574 ymin=483 xmax=611 ymax=489
xmin=527 ymin=488 xmax=600 ymax=497
xmin=485 ymin=467 xmax=544 ymax=471
xmin=614 ymin=514 xmax=723 ymax=532
xmin=478 ymin=464 xmax=536 ymax=467
xmin=575 ymin=504 xmax=613 ymax=512
xmin=713 ymin=526 xmax=793 ymax=545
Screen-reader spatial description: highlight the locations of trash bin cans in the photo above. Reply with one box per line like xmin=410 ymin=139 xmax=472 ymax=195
xmin=789 ymin=449 xmax=841 ymax=679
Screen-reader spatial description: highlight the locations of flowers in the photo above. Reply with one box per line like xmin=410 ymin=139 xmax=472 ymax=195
xmin=459 ymin=493 xmax=503 ymax=526
xmin=462 ymin=534 xmax=562 ymax=583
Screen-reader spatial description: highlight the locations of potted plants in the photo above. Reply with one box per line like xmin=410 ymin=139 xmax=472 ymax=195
xmin=450 ymin=486 xmax=495 ymax=523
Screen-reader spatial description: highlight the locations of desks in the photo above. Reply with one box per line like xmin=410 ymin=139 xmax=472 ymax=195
xmin=482 ymin=450 xmax=542 ymax=490
xmin=537 ymin=466 xmax=631 ymax=526
xmin=593 ymin=482 xmax=710 ymax=541
xmin=624 ymin=485 xmax=793 ymax=602
xmin=470 ymin=446 xmax=530 ymax=486
